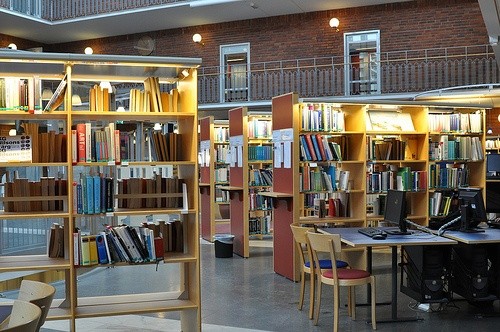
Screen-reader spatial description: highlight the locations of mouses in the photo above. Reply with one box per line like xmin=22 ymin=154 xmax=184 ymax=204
xmin=373 ymin=235 xmax=385 ymax=240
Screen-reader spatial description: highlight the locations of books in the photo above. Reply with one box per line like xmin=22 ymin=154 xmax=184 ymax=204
xmin=248 ymin=166 xmax=273 ymax=186
xmin=71 ymin=122 xmax=183 ymax=163
xmin=248 ymin=117 xmax=272 ymax=138
xmin=215 ymin=184 xmax=230 ymax=202
xmin=47 ymin=223 xmax=64 ymax=258
xmin=300 ymin=135 xmax=348 ymax=162
xmin=3 ymin=170 xmax=66 ymax=212
xmin=73 ymin=174 xmax=186 ymax=215
xmin=248 ymin=144 xmax=272 ymax=161
xmin=73 ymin=221 xmax=187 ymax=267
xmin=427 ymin=192 xmax=451 ymax=216
xmin=432 ymin=112 xmax=498 ymax=160
xmin=367 ymin=164 xmax=426 ymax=216
xmin=248 ymin=214 xmax=271 ymax=235
xmin=89 ymin=77 xmax=178 ymax=112
xmin=214 ymin=127 xmax=229 ymax=142
xmin=301 ymin=105 xmax=345 ymax=133
xmin=304 ymin=194 xmax=348 ymax=218
xmin=300 ymin=162 xmax=350 ymax=193
xmin=366 ymin=135 xmax=418 ymax=161
xmin=0 ymin=74 xmax=67 ymax=114
xmin=214 ymin=145 xmax=229 ymax=161
xmin=249 ymin=187 xmax=273 ymax=211
xmin=429 ymin=163 xmax=471 ymax=188
xmin=214 ymin=165 xmax=230 ymax=183
xmin=0 ymin=122 xmax=66 ymax=162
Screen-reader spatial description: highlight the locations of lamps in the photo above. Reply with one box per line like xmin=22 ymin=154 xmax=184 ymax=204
xmin=9 ymin=43 xmax=18 ymax=49
xmin=84 ymin=46 xmax=94 ymax=54
xmin=193 ymin=33 xmax=204 ymax=47
xmin=329 ymin=17 xmax=341 ymax=33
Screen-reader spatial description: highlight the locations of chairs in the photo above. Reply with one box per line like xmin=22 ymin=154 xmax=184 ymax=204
xmin=0 ymin=280 xmax=57 ymax=331
xmin=290 ymin=223 xmax=378 ymax=332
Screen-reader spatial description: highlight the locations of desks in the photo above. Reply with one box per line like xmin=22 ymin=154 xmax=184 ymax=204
xmin=318 ymin=227 xmax=458 ymax=323
xmin=430 ymin=226 xmax=500 ymax=318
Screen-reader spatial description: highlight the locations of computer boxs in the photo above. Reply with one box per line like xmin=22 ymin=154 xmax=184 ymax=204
xmin=450 ymin=245 xmax=489 ymax=297
xmin=405 ymin=245 xmax=449 ymax=298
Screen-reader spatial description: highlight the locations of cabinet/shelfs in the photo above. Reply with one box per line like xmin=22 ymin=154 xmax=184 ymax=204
xmin=272 ymin=93 xmax=500 ymax=282
xmin=0 ymin=50 xmax=203 ymax=332
xmin=221 ymin=107 xmax=272 ymax=258
xmin=199 ymin=116 xmax=231 ymax=243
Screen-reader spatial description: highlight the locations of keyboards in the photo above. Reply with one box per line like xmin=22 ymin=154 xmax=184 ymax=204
xmin=358 ymin=227 xmax=387 ymax=237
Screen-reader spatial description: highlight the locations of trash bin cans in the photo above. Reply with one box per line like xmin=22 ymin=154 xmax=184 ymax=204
xmin=212 ymin=234 xmax=235 ymax=259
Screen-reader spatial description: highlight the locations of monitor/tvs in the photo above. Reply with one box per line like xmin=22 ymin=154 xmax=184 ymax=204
xmin=456 ymin=187 xmax=488 ymax=233
xmin=383 ymin=190 xmax=412 ymax=235
